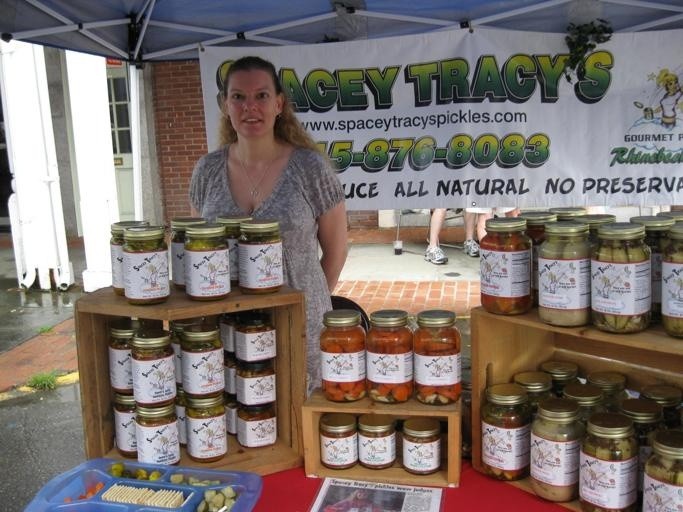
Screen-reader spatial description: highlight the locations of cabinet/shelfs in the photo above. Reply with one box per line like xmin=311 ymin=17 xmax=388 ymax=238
xmin=301 ymin=388 xmax=462 ymax=488
xmin=470 ymin=305 xmax=683 ymax=512
xmin=75 ymin=287 xmax=307 ymax=477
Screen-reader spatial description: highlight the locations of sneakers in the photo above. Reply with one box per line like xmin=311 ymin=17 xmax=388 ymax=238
xmin=423 ymin=245 xmax=449 ymax=266
xmin=463 ymin=239 xmax=480 ymax=258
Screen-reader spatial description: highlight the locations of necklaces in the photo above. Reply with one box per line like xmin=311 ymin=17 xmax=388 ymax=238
xmin=234 ymin=135 xmax=284 ymax=198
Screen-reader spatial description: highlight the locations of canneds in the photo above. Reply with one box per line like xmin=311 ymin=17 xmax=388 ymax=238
xmin=413 ymin=310 xmax=462 ymax=405
xmin=320 ymin=413 xmax=357 ymax=470
xmin=109 ymin=314 xmax=277 ymax=464
xmin=481 ymin=360 xmax=683 ymax=510
xmin=364 ymin=309 xmax=413 ymax=404
xmin=401 ymin=418 xmax=442 ymax=475
xmin=481 ymin=207 xmax=683 ymax=339
xmin=110 ymin=217 xmax=282 ymax=305
xmin=321 ymin=308 xmax=366 ymax=402
xmin=356 ymin=415 xmax=397 ymax=470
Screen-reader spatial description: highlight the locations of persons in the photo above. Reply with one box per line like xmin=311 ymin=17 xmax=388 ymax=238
xmin=322 ymin=488 xmax=382 ymax=512
xmin=424 ymin=208 xmax=481 ymax=264
xmin=476 ymin=207 xmax=519 ymax=243
xmin=643 ymin=68 xmax=683 ymax=130
xmin=189 ymin=55 xmax=350 ymax=401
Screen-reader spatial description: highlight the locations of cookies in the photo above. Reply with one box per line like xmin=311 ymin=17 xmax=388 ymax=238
xmin=101 ymin=482 xmax=193 ymax=509
